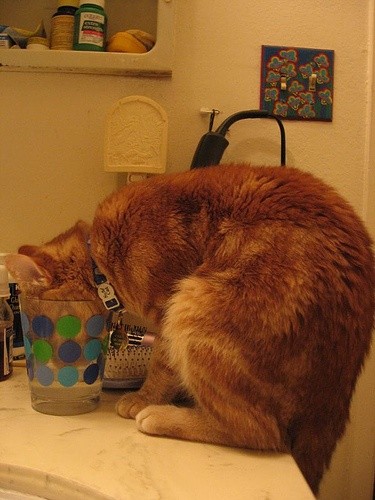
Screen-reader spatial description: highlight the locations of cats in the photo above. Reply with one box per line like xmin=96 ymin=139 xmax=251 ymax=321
xmin=4 ymin=161 xmax=374 ymax=498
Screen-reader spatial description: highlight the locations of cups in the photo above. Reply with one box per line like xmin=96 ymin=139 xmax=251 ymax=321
xmin=15 ymin=295 xmax=107 ymax=416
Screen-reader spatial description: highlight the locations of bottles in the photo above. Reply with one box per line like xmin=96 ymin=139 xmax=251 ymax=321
xmin=50 ymin=0 xmax=80 ymax=49
xmin=74 ymin=1 xmax=107 ymax=51
